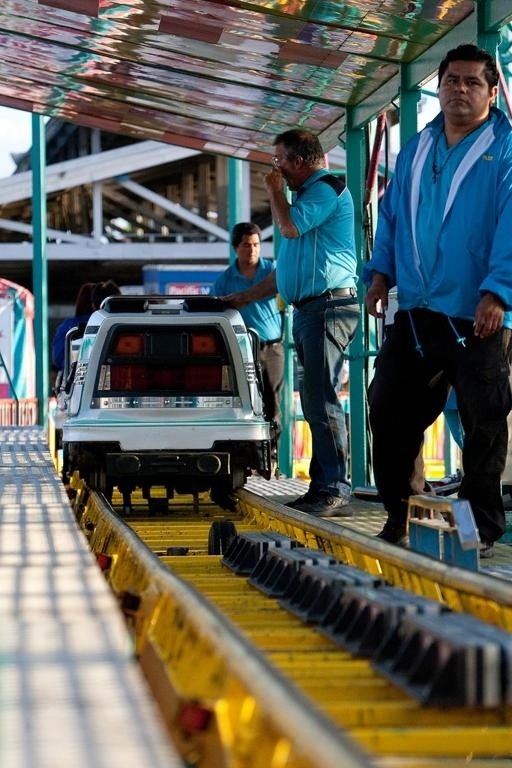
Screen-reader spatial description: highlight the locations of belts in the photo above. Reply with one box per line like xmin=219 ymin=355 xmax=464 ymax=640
xmin=289 ymin=288 xmax=355 ymax=309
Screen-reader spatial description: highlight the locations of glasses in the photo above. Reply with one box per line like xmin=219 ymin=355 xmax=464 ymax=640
xmin=271 ymin=155 xmax=284 ymax=169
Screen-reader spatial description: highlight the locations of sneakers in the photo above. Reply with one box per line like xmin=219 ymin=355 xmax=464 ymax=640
xmin=375 ymin=525 xmax=410 ymax=545
xmin=283 ymin=491 xmax=356 ymax=517
xmin=478 ymin=542 xmax=497 ymax=558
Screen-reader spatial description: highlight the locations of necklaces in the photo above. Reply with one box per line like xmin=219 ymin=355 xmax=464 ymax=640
xmin=430 ymin=127 xmax=479 ymax=184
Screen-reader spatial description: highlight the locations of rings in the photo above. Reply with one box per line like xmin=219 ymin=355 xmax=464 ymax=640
xmin=478 ymin=319 xmax=487 ymax=324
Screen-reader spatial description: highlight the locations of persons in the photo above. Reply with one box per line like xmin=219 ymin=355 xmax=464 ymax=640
xmin=91 ymin=281 xmax=122 ymax=311
xmin=55 ymin=282 xmax=97 ymax=372
xmin=215 ymin=130 xmax=361 ymax=514
xmin=207 ymin=221 xmax=284 ymax=477
xmin=364 ymin=44 xmax=512 ymax=560
